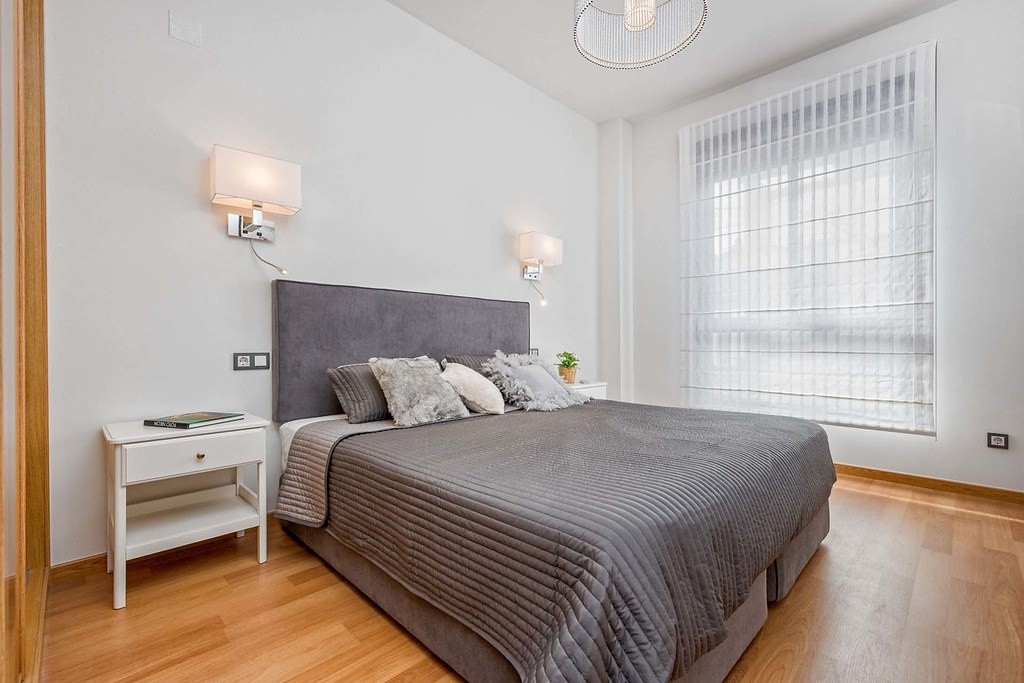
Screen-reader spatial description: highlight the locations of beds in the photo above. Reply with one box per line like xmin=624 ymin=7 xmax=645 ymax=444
xmin=272 ymin=279 xmax=837 ymax=683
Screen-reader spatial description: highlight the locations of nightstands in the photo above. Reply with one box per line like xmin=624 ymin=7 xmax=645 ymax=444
xmin=567 ymin=383 xmax=608 ymax=401
xmin=100 ymin=412 xmax=269 ymax=609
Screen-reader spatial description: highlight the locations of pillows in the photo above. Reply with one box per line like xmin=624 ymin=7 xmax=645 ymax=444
xmin=326 ymin=363 xmax=391 ymax=425
xmin=482 ymin=350 xmax=588 ymax=412
xmin=440 ymin=358 xmax=505 ymax=415
xmin=370 ymin=358 xmax=471 ymax=426
xmin=444 ymin=353 xmax=494 ymax=376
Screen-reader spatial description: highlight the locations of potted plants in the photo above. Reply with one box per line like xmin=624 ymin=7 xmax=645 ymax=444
xmin=555 ymin=351 xmax=579 ymax=383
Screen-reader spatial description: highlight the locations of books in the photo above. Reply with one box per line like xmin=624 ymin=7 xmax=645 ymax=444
xmin=144 ymin=412 xmax=245 ymax=429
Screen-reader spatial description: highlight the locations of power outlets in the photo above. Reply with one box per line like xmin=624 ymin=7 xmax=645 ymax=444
xmin=988 ymin=432 xmax=1008 ymax=449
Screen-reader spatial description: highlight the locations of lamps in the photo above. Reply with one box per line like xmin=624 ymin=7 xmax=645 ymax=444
xmin=520 ymin=232 xmax=564 ymax=280
xmin=573 ymin=0 xmax=710 ymax=71
xmin=209 ymin=144 xmax=302 ymax=241
